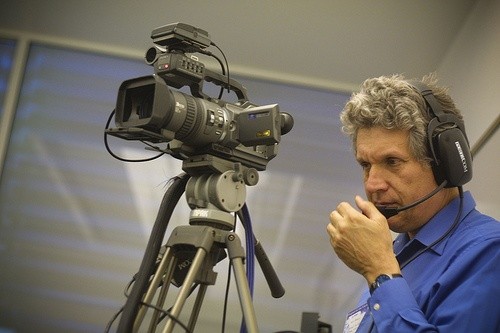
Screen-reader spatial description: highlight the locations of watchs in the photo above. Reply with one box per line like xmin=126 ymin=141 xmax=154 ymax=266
xmin=369 ymin=273 xmax=403 ymax=296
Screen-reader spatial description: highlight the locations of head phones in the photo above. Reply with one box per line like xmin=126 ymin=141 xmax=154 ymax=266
xmin=404 ymin=79 xmax=473 ymax=188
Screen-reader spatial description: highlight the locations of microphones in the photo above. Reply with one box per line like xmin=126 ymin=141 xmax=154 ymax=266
xmin=361 ymin=180 xmax=447 ymax=221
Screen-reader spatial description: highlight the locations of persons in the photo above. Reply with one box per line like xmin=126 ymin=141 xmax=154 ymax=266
xmin=327 ymin=71 xmax=499 ymax=333
